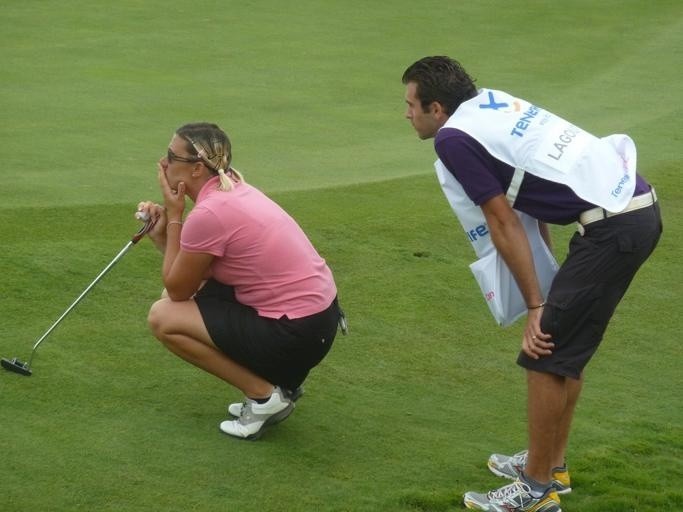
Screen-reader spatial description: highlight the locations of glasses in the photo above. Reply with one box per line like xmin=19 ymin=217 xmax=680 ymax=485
xmin=167 ymin=148 xmax=199 ymax=164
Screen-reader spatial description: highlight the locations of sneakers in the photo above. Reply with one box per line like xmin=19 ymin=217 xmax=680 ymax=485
xmin=487 ymin=449 xmax=572 ymax=494
xmin=462 ymin=471 xmax=561 ymax=512
xmin=219 ymin=385 xmax=305 ymax=439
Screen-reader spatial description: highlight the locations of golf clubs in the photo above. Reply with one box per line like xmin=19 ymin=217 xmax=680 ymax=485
xmin=0 ymin=214 xmax=161 ymax=375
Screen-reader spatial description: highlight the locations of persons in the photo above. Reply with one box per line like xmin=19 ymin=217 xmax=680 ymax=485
xmin=135 ymin=122 xmax=340 ymax=441
xmin=403 ymin=55 xmax=663 ymax=512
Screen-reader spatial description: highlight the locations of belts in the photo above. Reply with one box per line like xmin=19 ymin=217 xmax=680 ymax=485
xmin=573 ymin=186 xmax=657 ymax=238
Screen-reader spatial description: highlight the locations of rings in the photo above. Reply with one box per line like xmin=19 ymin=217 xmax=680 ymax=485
xmin=532 ymin=335 xmax=536 ymax=339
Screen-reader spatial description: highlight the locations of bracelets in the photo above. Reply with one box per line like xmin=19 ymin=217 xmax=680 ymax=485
xmin=527 ymin=302 xmax=545 ymax=309
xmin=167 ymin=221 xmax=182 ymax=227
